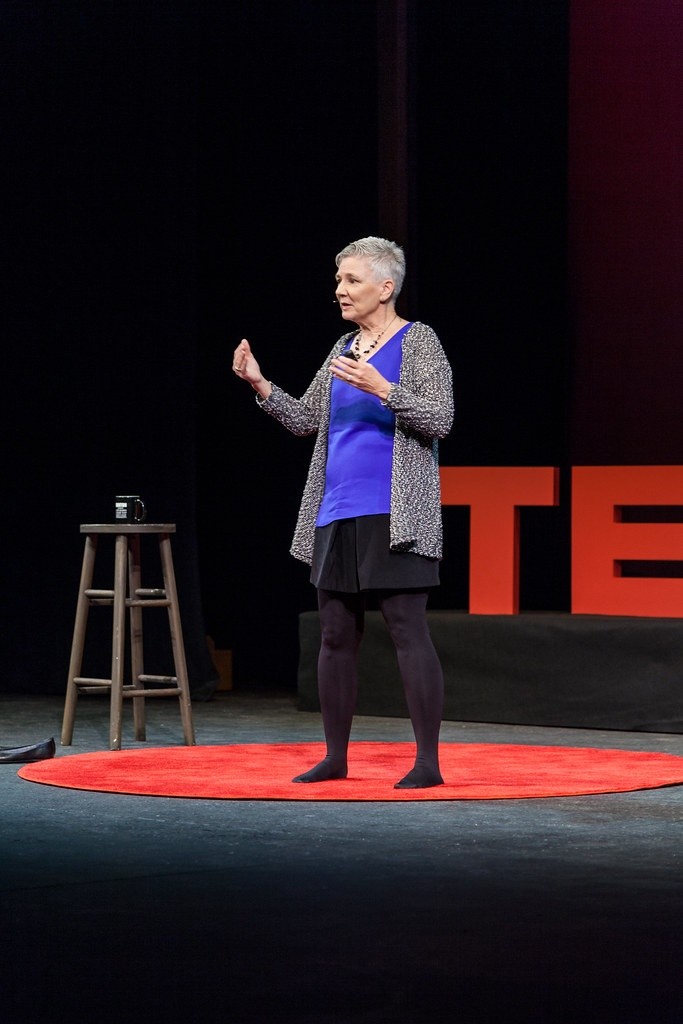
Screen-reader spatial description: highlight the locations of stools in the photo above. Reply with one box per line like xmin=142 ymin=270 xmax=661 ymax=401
xmin=57 ymin=520 xmax=194 ymax=750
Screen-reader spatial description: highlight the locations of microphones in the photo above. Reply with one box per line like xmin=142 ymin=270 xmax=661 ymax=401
xmin=333 ymin=300 xmax=338 ymax=303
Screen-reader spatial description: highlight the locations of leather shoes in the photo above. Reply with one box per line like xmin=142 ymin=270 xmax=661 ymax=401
xmin=0 ymin=736 xmax=56 ymax=764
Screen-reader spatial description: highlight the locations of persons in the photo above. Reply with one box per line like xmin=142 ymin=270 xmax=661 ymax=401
xmin=232 ymin=235 xmax=455 ymax=789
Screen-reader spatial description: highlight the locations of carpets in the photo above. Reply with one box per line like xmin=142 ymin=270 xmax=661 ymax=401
xmin=17 ymin=741 xmax=683 ymax=804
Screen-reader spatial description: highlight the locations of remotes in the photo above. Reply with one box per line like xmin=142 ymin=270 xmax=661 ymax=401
xmin=343 ymin=350 xmax=358 ymax=362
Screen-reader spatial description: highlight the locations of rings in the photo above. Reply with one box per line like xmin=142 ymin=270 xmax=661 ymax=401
xmin=235 ymin=366 xmax=241 ymax=371
xmin=347 ymin=375 xmax=352 ymax=381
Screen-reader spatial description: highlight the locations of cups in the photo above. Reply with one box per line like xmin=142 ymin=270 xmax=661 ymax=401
xmin=115 ymin=495 xmax=146 ymax=524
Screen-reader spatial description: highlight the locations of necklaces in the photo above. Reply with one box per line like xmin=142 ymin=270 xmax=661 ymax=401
xmin=355 ymin=314 xmax=397 ymax=360
